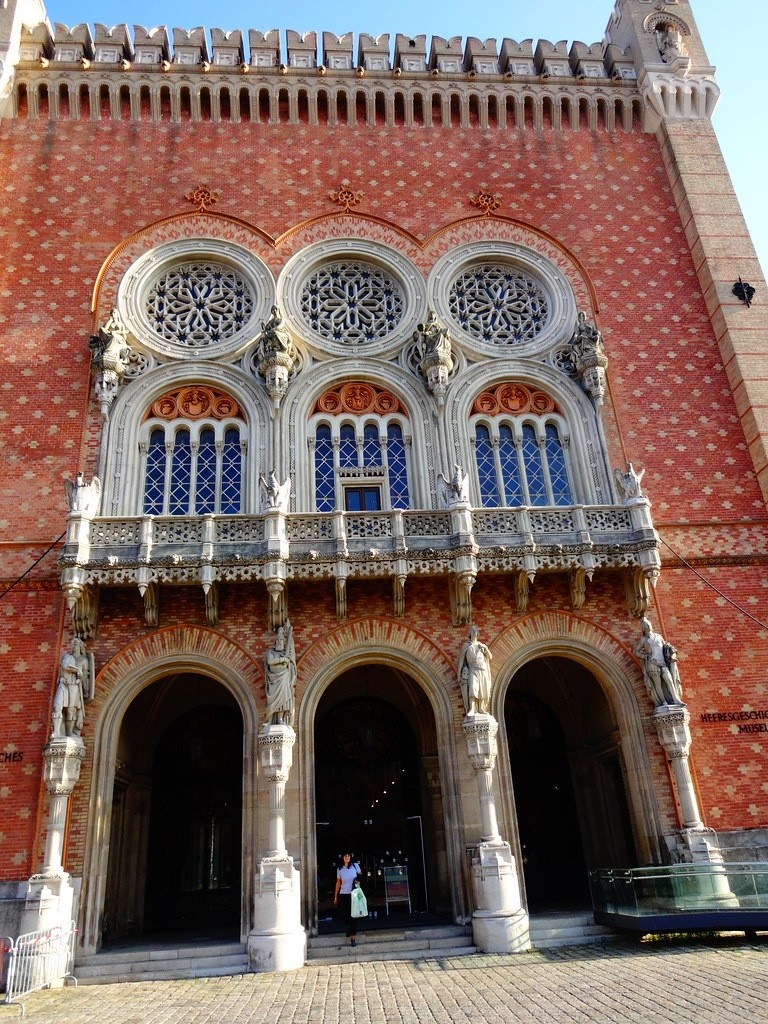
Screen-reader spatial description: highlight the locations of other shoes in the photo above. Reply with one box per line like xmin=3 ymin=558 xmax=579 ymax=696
xmin=351 ymin=938 xmax=357 ymax=947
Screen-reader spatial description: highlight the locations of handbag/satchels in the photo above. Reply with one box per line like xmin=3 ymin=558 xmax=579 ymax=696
xmin=350 ymin=883 xmax=368 ymax=918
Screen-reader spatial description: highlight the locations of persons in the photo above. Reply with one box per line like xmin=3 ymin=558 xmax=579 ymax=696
xmin=414 ymin=310 xmax=452 ymax=356
xmin=262 ymin=626 xmax=296 ymax=726
xmin=259 ymin=305 xmax=293 ymax=355
xmin=49 ymin=638 xmax=88 ymax=740
xmin=457 ymin=625 xmax=492 ymax=716
xmin=568 ymin=311 xmax=601 ymax=362
xmin=333 ymin=849 xmax=363 ymax=947
xmin=94 ymin=309 xmax=130 ymax=360
xmin=634 ymin=620 xmax=687 ymax=706
xmin=660 ymin=25 xmax=689 ymax=62
